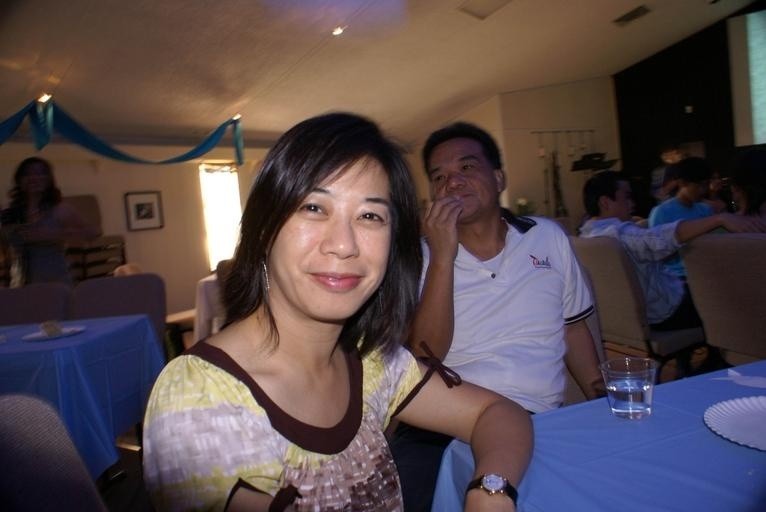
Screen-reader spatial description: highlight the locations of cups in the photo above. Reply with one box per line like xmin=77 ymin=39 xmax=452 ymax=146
xmin=600 ymin=358 xmax=658 ymax=420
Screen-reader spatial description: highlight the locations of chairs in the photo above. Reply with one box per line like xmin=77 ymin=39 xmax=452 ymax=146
xmin=0 ymin=394 xmax=106 ymax=512
xmin=0 ymin=273 xmax=166 ymax=325
xmin=563 ymin=266 xmax=607 ymax=407
xmin=571 ymin=235 xmax=703 ymax=381
xmin=685 ymin=233 xmax=766 ymax=366
xmin=193 ymin=273 xmax=228 ymax=344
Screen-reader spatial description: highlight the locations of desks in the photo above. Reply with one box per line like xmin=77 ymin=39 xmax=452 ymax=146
xmin=432 ymin=360 xmax=766 ymax=512
xmin=0 ymin=314 xmax=164 ymax=497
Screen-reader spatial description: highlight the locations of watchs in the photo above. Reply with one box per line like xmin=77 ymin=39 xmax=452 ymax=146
xmin=465 ymin=472 xmax=518 ymax=507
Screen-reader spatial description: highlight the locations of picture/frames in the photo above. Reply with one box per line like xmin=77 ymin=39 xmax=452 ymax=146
xmin=125 ymin=191 xmax=164 ymax=231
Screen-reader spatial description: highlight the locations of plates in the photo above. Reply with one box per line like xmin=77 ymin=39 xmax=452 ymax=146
xmin=703 ymin=395 xmax=766 ymax=453
xmin=22 ymin=327 xmax=84 ymax=341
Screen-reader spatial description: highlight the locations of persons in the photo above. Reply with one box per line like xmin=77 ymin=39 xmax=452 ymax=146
xmin=396 ymin=122 xmax=608 ymax=417
xmin=1 ymin=157 xmax=84 ymax=281
xmin=141 ymin=113 xmax=534 ymax=512
